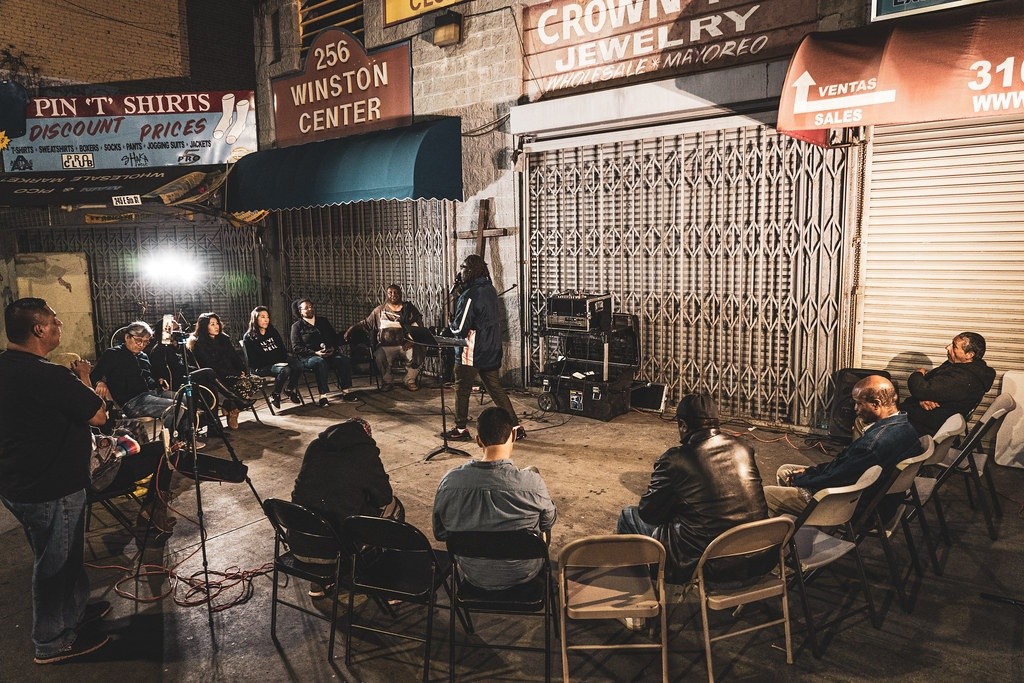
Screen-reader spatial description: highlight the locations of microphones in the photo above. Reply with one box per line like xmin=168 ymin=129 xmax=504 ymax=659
xmin=449 ymin=283 xmax=458 ymax=294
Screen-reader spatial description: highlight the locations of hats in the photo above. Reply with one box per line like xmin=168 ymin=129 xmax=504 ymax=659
xmin=676 ymin=393 xmax=718 ymax=419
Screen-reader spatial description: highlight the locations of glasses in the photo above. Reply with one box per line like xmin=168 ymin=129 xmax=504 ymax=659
xmin=129 ymin=333 xmax=150 ymax=346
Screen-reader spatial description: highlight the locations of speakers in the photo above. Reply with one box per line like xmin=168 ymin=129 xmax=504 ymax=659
xmin=828 ymin=368 xmax=891 ymax=438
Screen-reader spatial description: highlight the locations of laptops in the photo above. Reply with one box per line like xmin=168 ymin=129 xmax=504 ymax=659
xmin=403 ymin=324 xmax=451 ymax=345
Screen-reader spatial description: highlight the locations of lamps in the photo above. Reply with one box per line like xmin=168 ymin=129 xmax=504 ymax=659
xmin=434 ymin=9 xmax=463 ymax=49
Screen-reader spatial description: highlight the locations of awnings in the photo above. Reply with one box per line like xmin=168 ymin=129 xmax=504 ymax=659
xmin=227 ymin=116 xmax=463 ymax=212
xmin=777 ymin=0 xmax=1024 ymax=148
xmin=0 ymin=164 xmax=235 ymax=206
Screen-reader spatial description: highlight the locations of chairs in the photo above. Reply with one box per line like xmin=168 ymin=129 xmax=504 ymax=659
xmin=86 ymin=325 xmax=1016 ymax=683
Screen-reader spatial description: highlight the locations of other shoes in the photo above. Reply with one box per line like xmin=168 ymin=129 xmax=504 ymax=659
xmin=402 ymin=380 xmax=418 ymax=392
xmin=380 ymin=383 xmax=394 ymax=392
xmin=190 ymin=440 xmax=206 ymax=450
xmin=440 ymin=428 xmax=472 ymax=442
xmin=136 ymin=513 xmax=177 ymax=534
xmin=227 ymin=409 xmax=240 ymax=430
xmin=342 ymin=392 xmax=358 ymax=402
xmin=308 ymin=582 xmax=335 ymax=598
xmin=615 ymin=617 xmax=646 ymax=631
xmin=283 ymin=388 xmax=300 ymax=404
xmin=516 ymin=426 xmax=527 ymax=440
xmin=34 ymin=601 xmax=111 ymax=664
xmin=270 ymin=392 xmax=281 ymax=409
xmin=318 ymin=398 xmax=329 ymax=407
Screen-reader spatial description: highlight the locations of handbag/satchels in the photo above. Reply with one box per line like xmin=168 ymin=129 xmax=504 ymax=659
xmin=88 ymin=433 xmax=122 ymax=493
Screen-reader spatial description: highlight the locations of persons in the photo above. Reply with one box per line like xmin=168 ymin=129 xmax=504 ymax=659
xmin=186 ymin=312 xmax=243 ymax=430
xmin=431 ymin=407 xmax=557 ymax=590
xmin=854 ymin=332 xmax=996 ymax=442
xmin=343 ymin=285 xmax=424 ymax=392
xmin=149 ymin=318 xmax=257 ymax=437
xmin=616 ymin=394 xmax=779 ymax=630
xmin=243 ymin=305 xmax=300 ymax=408
xmin=0 ymin=297 xmax=113 ymax=664
xmin=45 ymin=353 xmax=177 ymax=541
xmin=89 ymin=321 xmax=205 ymax=449
xmin=291 ymin=416 xmax=405 ymax=604
xmin=446 ymin=255 xmax=527 ymax=441
xmin=763 ymin=374 xmax=924 ymax=526
xmin=290 ymin=297 xmax=360 ymax=408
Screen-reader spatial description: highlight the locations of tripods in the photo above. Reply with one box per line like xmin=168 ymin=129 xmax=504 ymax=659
xmin=405 ymin=340 xmax=471 ymax=460
xmin=134 ymin=340 xmax=287 ymax=587
